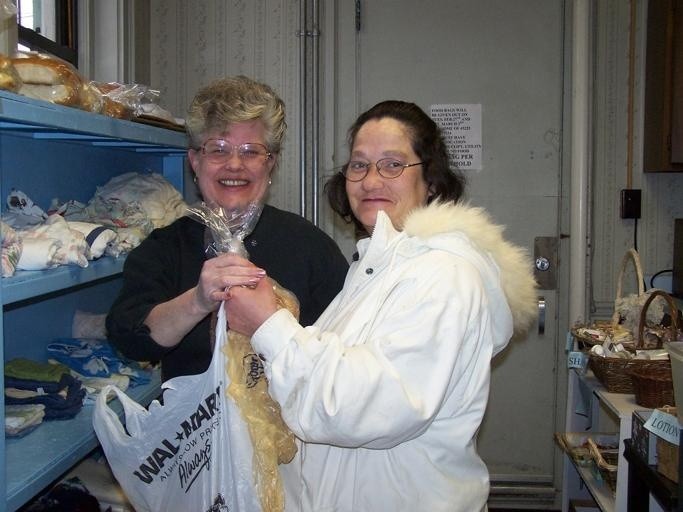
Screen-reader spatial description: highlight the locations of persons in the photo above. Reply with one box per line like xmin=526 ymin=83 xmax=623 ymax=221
xmin=106 ymin=82 xmax=349 ymax=393
xmin=219 ymin=99 xmax=539 ymax=512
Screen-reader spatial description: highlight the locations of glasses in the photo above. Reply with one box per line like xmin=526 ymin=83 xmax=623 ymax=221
xmin=340 ymin=158 xmax=423 ymax=183
xmin=198 ymin=138 xmax=272 ymax=168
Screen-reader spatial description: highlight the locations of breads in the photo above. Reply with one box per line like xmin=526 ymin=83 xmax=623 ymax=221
xmin=0 ymin=45 xmax=128 ymax=119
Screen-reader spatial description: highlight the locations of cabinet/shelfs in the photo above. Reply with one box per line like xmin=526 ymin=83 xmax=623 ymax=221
xmin=559 ymin=359 xmax=682 ymax=511
xmin=0 ymin=90 xmax=193 ymax=512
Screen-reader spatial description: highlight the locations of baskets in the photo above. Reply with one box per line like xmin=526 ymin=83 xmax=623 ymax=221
xmin=563 ymin=431 xmax=619 ymax=491
xmin=571 ymin=248 xmax=683 ymax=407
